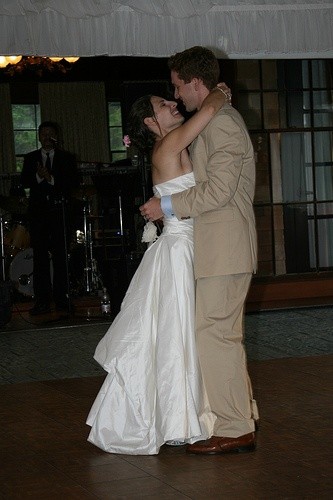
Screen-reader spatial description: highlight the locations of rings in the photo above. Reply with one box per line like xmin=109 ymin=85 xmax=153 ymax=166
xmin=144 ymin=215 xmax=148 ymax=221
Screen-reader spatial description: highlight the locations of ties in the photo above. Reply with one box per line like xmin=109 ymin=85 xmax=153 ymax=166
xmin=44 ymin=152 xmax=51 ymax=169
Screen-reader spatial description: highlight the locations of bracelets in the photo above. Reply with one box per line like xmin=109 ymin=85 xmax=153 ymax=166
xmin=211 ymin=87 xmax=231 ymax=107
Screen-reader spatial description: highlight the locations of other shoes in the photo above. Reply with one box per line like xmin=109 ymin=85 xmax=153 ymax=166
xmin=28 ymin=303 xmax=50 ymax=315
xmin=55 ymin=300 xmax=76 ymax=313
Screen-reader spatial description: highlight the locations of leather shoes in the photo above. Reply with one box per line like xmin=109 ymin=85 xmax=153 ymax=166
xmin=186 ymin=430 xmax=254 ymax=454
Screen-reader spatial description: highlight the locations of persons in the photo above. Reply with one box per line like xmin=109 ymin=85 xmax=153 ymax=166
xmin=20 ymin=121 xmax=80 ymax=316
xmin=123 ymin=46 xmax=259 ymax=460
xmin=82 ymin=85 xmax=233 ymax=455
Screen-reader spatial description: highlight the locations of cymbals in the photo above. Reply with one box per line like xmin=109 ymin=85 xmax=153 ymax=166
xmin=83 ymin=210 xmax=106 ymax=219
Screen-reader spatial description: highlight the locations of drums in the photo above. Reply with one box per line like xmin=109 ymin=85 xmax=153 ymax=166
xmin=5 ymin=219 xmax=33 ymax=252
xmin=8 ymin=246 xmax=56 ymax=298
